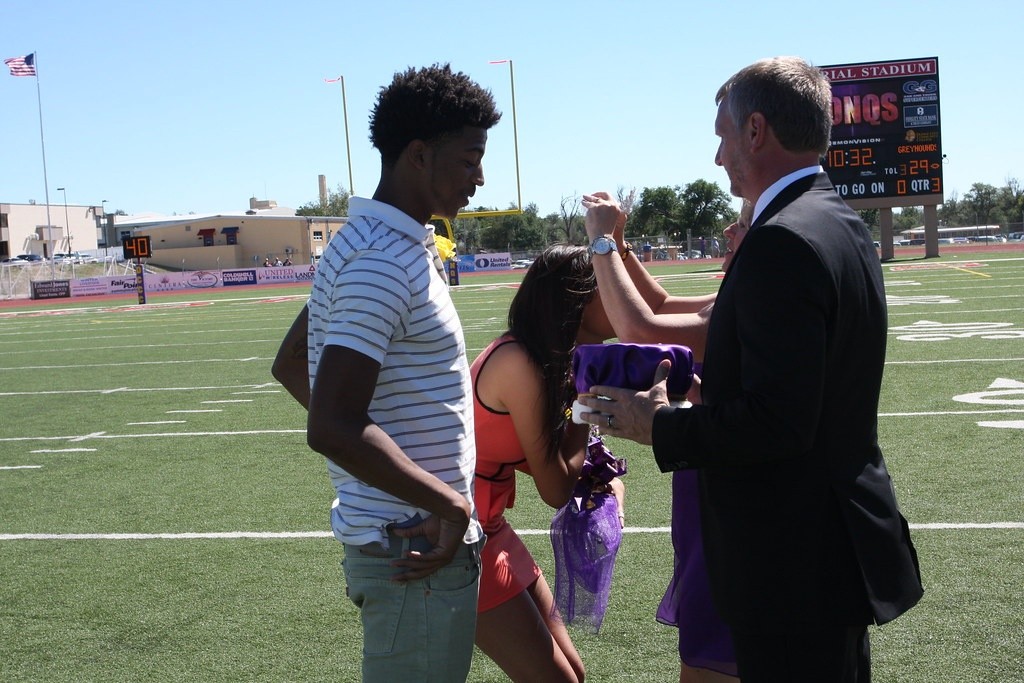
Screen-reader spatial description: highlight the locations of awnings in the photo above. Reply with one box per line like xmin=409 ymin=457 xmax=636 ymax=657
xmin=197 ymin=229 xmax=216 ymax=236
xmin=221 ymin=227 xmax=238 ymax=234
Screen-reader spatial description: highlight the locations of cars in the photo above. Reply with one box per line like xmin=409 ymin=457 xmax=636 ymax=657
xmin=635 ymin=245 xmax=711 ymax=262
xmin=0 ymin=250 xmax=100 ymax=264
xmin=874 ymin=231 xmax=1024 ymax=248
xmin=511 ymin=257 xmax=535 ymax=270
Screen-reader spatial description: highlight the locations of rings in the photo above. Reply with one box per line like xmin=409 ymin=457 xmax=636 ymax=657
xmin=618 ymin=512 xmax=624 ymax=518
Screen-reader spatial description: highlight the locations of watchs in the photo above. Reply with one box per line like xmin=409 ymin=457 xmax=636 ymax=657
xmin=587 ymin=235 xmax=617 ymax=255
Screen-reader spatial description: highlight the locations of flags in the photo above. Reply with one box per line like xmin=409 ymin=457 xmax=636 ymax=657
xmin=4 ymin=52 xmax=36 ymax=77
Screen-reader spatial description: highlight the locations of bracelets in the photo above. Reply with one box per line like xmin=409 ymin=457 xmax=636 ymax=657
xmin=608 ymin=416 xmax=613 ymax=427
xmin=622 ymin=240 xmax=634 ymax=260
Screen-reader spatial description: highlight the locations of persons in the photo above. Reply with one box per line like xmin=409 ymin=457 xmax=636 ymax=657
xmin=468 ymin=245 xmax=617 ymax=683
xmin=581 ymin=192 xmax=755 ymax=683
xmin=264 ymin=258 xmax=272 ymax=267
xmin=273 ymin=258 xmax=283 ymax=266
xmin=271 ymin=65 xmax=503 ymax=683
xmin=700 ymin=235 xmax=707 ymax=258
xmin=711 ymin=237 xmax=720 ymax=258
xmin=283 ymin=258 xmax=292 ymax=266
xmin=576 ymin=54 xmax=926 ymax=683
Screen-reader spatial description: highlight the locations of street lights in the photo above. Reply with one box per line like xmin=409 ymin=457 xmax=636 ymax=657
xmin=101 ymin=199 xmax=110 ymax=269
xmin=57 ymin=187 xmax=72 ymax=260
